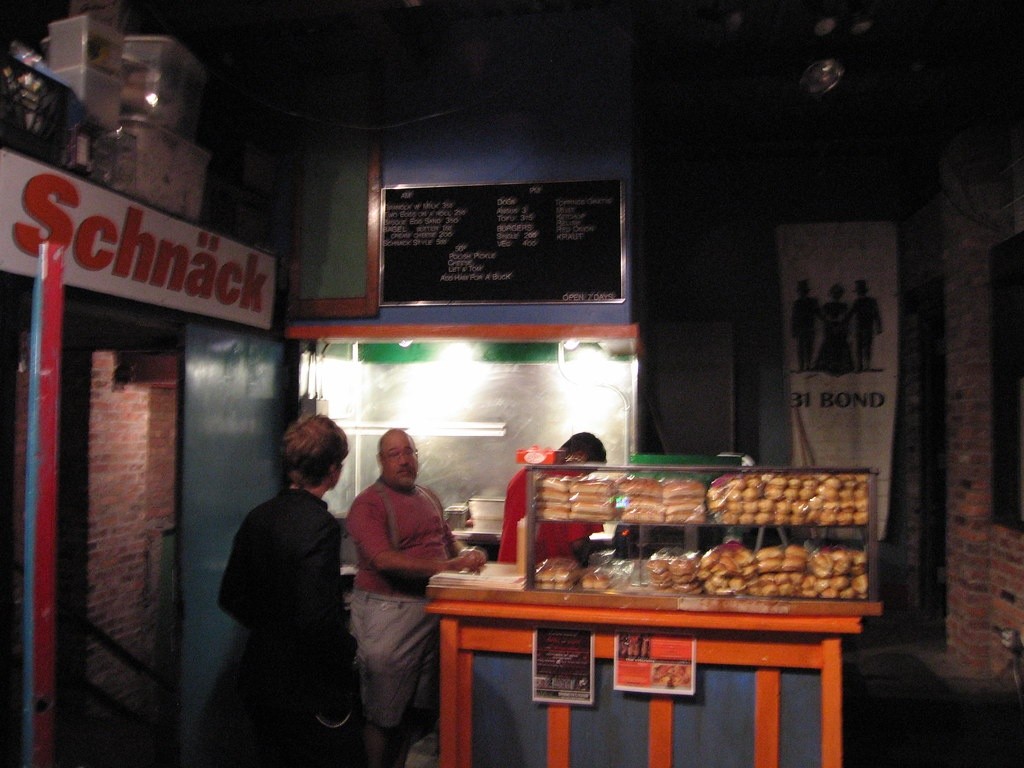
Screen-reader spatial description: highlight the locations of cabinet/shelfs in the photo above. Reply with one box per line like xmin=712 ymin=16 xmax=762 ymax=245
xmin=525 ymin=462 xmax=886 ymax=619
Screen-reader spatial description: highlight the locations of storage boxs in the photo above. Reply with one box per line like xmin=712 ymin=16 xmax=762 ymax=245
xmin=0 ymin=15 xmax=212 ymax=222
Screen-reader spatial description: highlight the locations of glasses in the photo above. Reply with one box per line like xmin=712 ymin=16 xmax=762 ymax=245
xmin=384 ymin=447 xmax=416 ymax=461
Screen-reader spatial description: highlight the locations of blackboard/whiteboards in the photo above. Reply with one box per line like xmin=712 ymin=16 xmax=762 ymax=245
xmin=379 ymin=178 xmax=626 ymax=305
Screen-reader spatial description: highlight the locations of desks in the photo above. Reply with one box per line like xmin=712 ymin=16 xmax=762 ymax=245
xmin=423 ymin=598 xmax=864 ymax=768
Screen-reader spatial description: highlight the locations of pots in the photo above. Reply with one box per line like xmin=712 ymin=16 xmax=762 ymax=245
xmin=468 ymin=498 xmax=505 ymax=529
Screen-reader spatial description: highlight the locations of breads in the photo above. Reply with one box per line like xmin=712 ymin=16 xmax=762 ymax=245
xmin=533 ymin=471 xmax=868 ymax=600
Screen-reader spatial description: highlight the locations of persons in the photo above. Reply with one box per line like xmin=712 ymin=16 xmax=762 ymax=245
xmin=346 ymin=428 xmax=486 ymax=768
xmin=498 ymin=433 xmax=607 ymax=565
xmin=217 ymin=414 xmax=371 ymax=768
